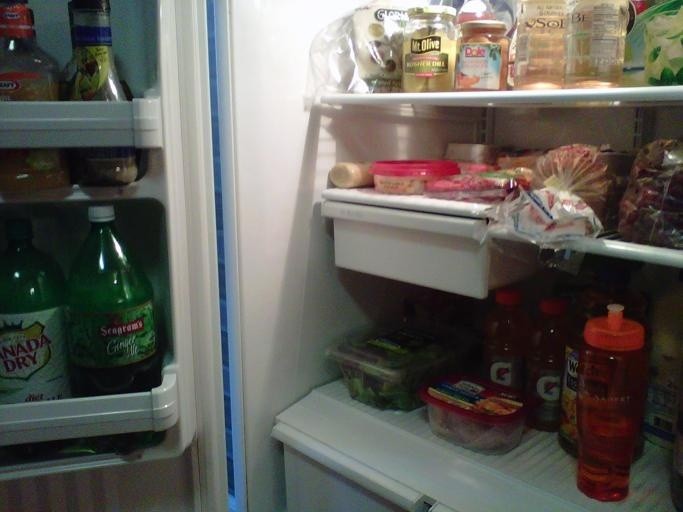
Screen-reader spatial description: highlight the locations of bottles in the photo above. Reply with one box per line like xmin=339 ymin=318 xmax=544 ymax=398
xmin=1 ymin=215 xmax=84 ymax=453
xmin=485 ymin=288 xmax=644 ymax=502
xmin=513 ymin=0 xmax=630 ymax=90
xmin=59 ymin=2 xmax=136 ymax=185
xmin=0 ymin=0 xmax=74 ymax=203
xmin=66 ymin=207 xmax=170 ymax=454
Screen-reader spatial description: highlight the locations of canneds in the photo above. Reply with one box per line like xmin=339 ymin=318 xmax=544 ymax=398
xmin=455 ymin=19 xmax=509 ymax=91
xmin=568 ymin=0 xmax=630 ymax=87
xmin=513 ymin=1 xmax=568 ymax=89
xmin=401 ymin=5 xmax=457 ymax=93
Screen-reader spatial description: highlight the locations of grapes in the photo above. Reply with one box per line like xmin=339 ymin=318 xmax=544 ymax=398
xmin=617 ymin=139 xmax=683 ymax=250
